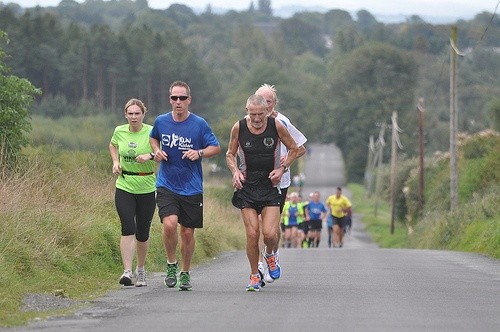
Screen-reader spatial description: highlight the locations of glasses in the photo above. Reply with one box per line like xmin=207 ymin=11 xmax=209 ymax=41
xmin=170 ymin=96 xmax=189 ymax=100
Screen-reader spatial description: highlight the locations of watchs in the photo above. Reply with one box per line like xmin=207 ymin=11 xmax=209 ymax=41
xmin=198 ymin=150 xmax=204 ymax=159
xmin=150 ymin=152 xmax=155 ymax=161
xmin=282 ymin=164 xmax=289 ymax=173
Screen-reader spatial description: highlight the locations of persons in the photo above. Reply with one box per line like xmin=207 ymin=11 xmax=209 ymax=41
xmin=278 ymin=187 xmax=354 ymax=250
xmin=108 ymin=98 xmax=160 ymax=287
xmin=226 ymin=94 xmax=299 ymax=291
xmin=235 ymin=83 xmax=308 ymax=282
xmin=148 ymin=81 xmax=222 ymax=291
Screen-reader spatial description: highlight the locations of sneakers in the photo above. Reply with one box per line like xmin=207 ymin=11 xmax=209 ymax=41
xmin=119 ymin=269 xmax=134 ymax=286
xmin=264 ymin=266 xmax=274 ymax=283
xmin=245 ymin=273 xmax=261 ymax=291
xmin=134 ymin=267 xmax=147 ymax=287
xmin=164 ymin=259 xmax=179 ymax=288
xmin=178 ymin=269 xmax=193 ymax=291
xmin=258 ymin=264 xmax=266 ymax=287
xmin=264 ymin=250 xmax=281 ymax=279
